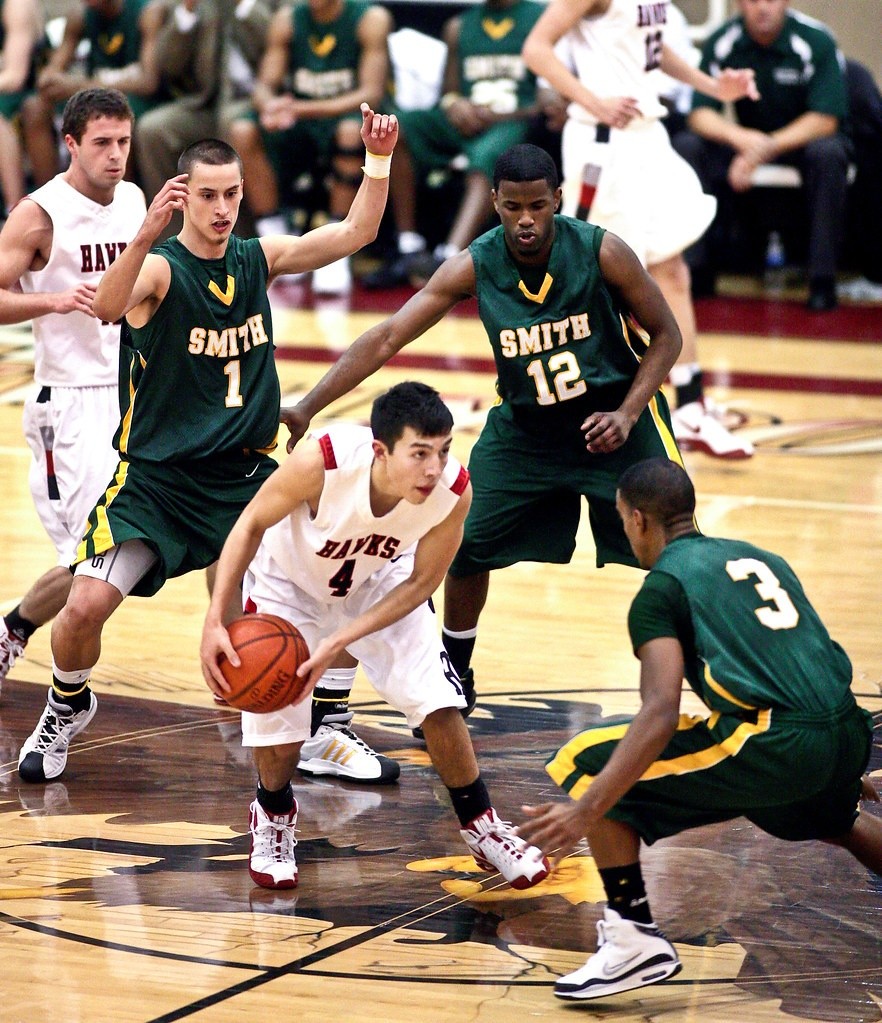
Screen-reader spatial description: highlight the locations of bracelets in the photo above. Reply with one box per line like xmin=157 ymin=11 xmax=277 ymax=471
xmin=360 ymin=148 xmax=393 ymax=179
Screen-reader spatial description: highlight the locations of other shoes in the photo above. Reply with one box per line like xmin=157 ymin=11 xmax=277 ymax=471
xmin=357 ymin=249 xmax=446 ymax=293
xmin=806 ymin=273 xmax=836 ymax=310
xmin=691 ymin=264 xmax=718 ymax=295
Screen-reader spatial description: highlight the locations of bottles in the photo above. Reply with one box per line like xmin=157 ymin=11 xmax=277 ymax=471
xmin=766 ymin=233 xmax=787 ymax=291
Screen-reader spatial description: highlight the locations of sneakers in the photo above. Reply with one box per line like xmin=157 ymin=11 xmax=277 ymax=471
xmin=296 ymin=710 xmax=400 ymax=783
xmin=0 ymin=615 xmax=28 ymax=683
xmin=460 ymin=807 xmax=550 ymax=891
xmin=18 ymin=687 xmax=97 ymax=783
xmin=411 ymin=664 xmax=479 ymax=747
xmin=550 ymin=907 xmax=682 ymax=1000
xmin=248 ymin=797 xmax=301 ymax=889
xmin=670 ymin=393 xmax=754 ymax=459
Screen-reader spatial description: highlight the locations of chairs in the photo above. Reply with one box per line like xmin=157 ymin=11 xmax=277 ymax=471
xmin=715 ymin=57 xmax=882 ymax=287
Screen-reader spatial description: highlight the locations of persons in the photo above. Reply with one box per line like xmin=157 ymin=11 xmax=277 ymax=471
xmin=17 ymin=102 xmax=400 ymax=782
xmin=507 ymin=460 xmax=882 ymax=1000
xmin=281 ymin=144 xmax=701 ymax=739
xmin=200 ymin=382 xmax=552 ymax=891
xmin=0 ymin=0 xmax=851 ymax=313
xmin=520 ymin=1 xmax=760 ymax=458
xmin=0 ymin=87 xmax=146 ymax=679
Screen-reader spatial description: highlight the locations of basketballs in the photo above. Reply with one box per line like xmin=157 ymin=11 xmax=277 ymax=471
xmin=214 ymin=612 xmax=312 ymax=714
